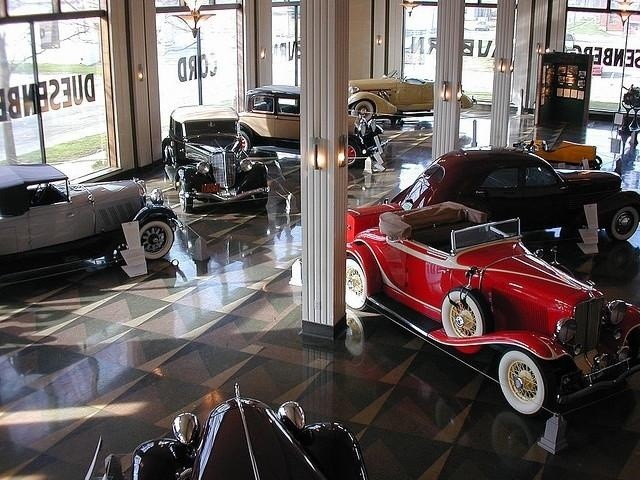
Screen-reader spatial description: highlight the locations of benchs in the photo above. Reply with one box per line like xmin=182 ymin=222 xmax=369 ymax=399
xmin=404 ymin=215 xmax=488 ymax=249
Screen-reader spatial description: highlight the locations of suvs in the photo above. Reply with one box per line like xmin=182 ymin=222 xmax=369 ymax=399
xmin=235 ymin=82 xmax=388 ymax=171
xmin=161 ymin=102 xmax=273 ymax=219
xmin=0 ymin=158 xmax=180 ymax=267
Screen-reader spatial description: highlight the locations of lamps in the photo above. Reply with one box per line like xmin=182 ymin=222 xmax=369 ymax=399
xmin=261 ymin=48 xmax=266 ymax=59
xmin=497 ymin=56 xmax=508 ymax=75
xmin=338 ymin=131 xmax=349 ymax=168
xmin=307 ymin=132 xmax=327 ymax=172
xmin=172 ymin=0 xmax=217 ymax=38
xmin=456 ymin=82 xmax=465 ymax=101
xmin=439 ymin=79 xmax=450 ymax=100
xmin=510 ymin=56 xmax=515 ymax=73
xmin=612 ymin=0 xmax=636 ymax=25
xmin=399 ymin=1 xmax=422 ymax=17
xmin=536 ymin=43 xmax=540 ymax=59
xmin=374 ymin=33 xmax=385 ymax=48
xmin=137 ymin=62 xmax=145 ymax=83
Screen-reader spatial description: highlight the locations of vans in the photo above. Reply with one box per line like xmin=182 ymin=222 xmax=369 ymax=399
xmin=592 ymin=64 xmax=603 ymax=75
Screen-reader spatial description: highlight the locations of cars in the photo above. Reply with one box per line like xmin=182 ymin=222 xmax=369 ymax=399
xmin=344 ymin=143 xmax=640 ymax=254
xmin=345 ymin=198 xmax=639 ymax=414
xmin=565 ymin=33 xmax=574 ymax=51
xmin=99 ymin=380 xmax=370 ymax=480
xmin=346 ymin=68 xmax=478 ymax=120
xmin=473 ymin=21 xmax=489 ymax=31
